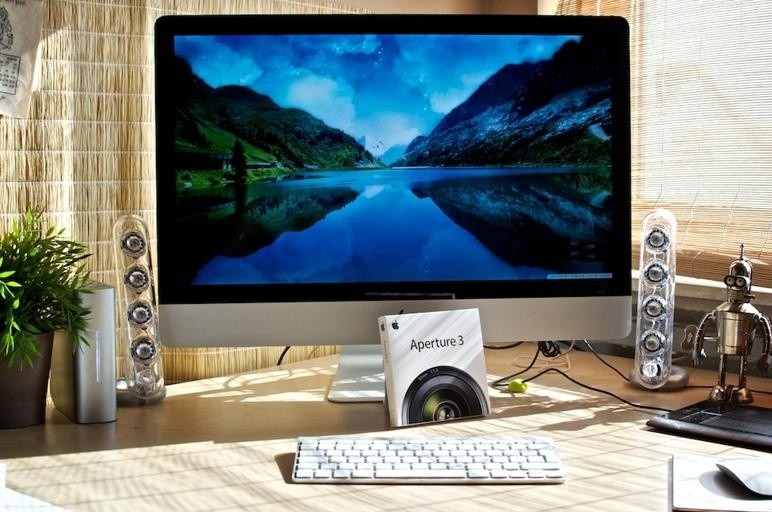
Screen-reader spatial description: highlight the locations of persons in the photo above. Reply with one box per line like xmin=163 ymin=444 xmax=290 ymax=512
xmin=680 ymin=242 xmax=768 ymax=403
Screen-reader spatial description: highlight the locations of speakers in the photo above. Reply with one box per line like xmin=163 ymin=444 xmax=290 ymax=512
xmin=112 ymin=212 xmax=168 ymax=407
xmin=628 ymin=208 xmax=690 ymax=391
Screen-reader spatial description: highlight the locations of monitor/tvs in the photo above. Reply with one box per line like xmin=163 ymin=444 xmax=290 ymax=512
xmin=154 ymin=16 xmax=632 ymax=406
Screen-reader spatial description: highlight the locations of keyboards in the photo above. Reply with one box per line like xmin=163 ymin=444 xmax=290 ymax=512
xmin=291 ymin=435 xmax=567 ymax=485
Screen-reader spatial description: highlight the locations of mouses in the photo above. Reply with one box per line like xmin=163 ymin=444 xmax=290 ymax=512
xmin=716 ymin=456 xmax=768 ymax=496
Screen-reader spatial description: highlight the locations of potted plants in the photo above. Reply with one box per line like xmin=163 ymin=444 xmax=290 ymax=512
xmin=0 ymin=188 xmax=95 ymax=429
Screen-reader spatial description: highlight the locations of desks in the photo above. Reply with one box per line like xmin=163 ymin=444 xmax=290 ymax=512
xmin=0 ymin=344 xmax=772 ymax=512
